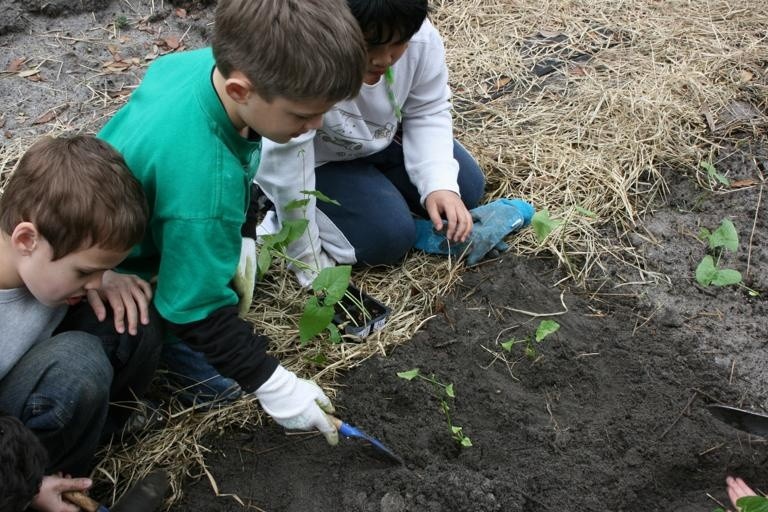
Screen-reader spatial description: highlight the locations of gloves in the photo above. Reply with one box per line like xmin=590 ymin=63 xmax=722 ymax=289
xmin=250 ymin=363 xmax=341 ymax=448
xmin=409 ymin=197 xmax=536 ymax=269
xmin=231 ymin=237 xmax=259 ymax=321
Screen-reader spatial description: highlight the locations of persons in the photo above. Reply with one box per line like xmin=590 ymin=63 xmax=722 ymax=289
xmin=0 ymin=133 xmax=164 ymax=512
xmin=93 ymin=2 xmax=366 ymax=447
xmin=251 ymin=2 xmax=485 ymax=293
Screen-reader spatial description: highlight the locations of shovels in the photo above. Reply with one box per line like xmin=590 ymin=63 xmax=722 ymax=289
xmin=325 ymin=414 xmax=403 ymax=465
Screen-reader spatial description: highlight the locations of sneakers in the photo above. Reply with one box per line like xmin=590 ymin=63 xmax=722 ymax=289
xmin=168 ymin=349 xmax=243 ymax=412
